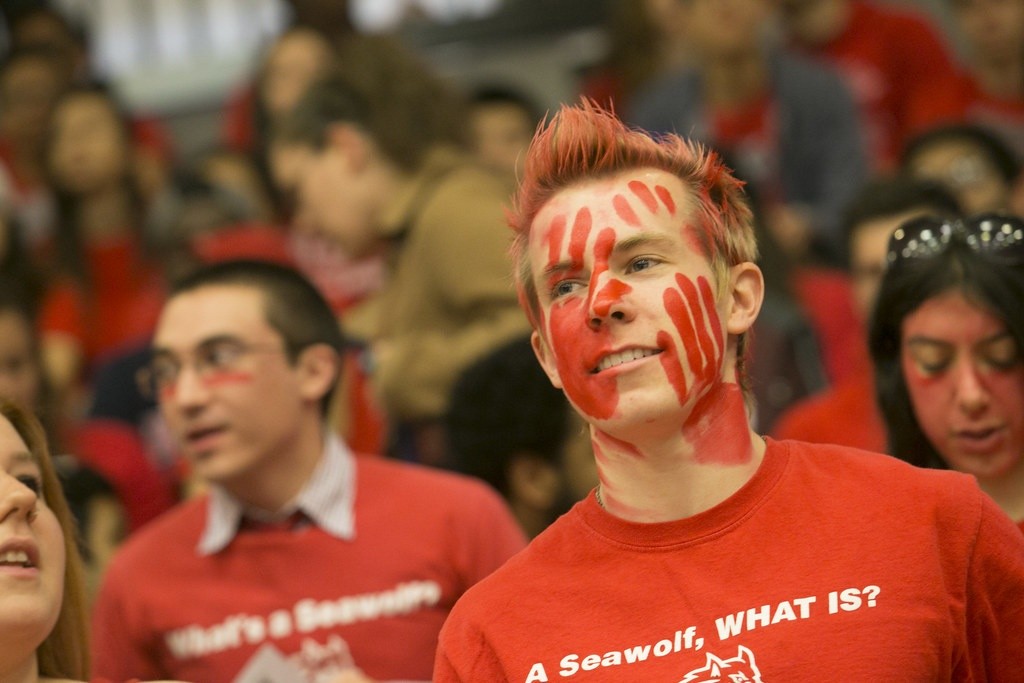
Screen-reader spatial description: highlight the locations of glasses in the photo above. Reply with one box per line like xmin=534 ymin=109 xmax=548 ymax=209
xmin=885 ymin=214 xmax=1024 ymax=282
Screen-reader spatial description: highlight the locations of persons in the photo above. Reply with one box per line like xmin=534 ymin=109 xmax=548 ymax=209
xmin=0 ymin=394 xmax=92 ymax=683
xmin=93 ymin=252 xmax=526 ymax=683
xmin=0 ymin=0 xmax=1024 ymax=579
xmin=430 ymin=108 xmax=1021 ymax=683
